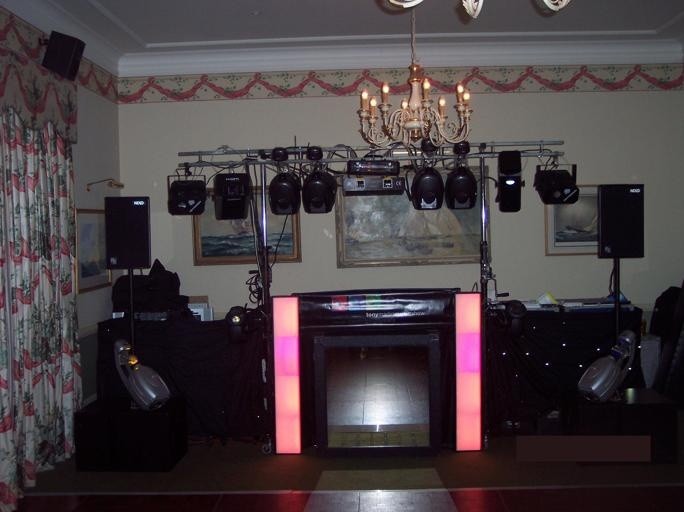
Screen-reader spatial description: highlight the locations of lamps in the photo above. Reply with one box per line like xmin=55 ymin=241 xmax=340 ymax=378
xmin=356 ymin=6 xmax=473 ymax=150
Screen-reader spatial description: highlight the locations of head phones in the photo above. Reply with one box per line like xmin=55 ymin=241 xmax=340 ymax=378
xmin=484 ymin=300 xmax=527 ymax=319
xmin=226 ymin=306 xmax=269 ymax=327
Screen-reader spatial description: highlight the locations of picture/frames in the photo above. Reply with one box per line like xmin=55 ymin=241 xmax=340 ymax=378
xmin=190 ymin=185 xmax=302 ymax=266
xmin=331 ymin=165 xmax=493 ymax=269
xmin=543 ymin=184 xmax=599 ymax=256
xmin=74 ymin=208 xmax=111 ymax=296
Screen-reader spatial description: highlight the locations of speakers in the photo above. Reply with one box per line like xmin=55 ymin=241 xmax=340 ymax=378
xmin=42 ymin=31 xmax=86 ymax=80
xmin=104 ymin=197 xmax=152 ymax=270
xmin=597 ymin=184 xmax=645 ymax=258
